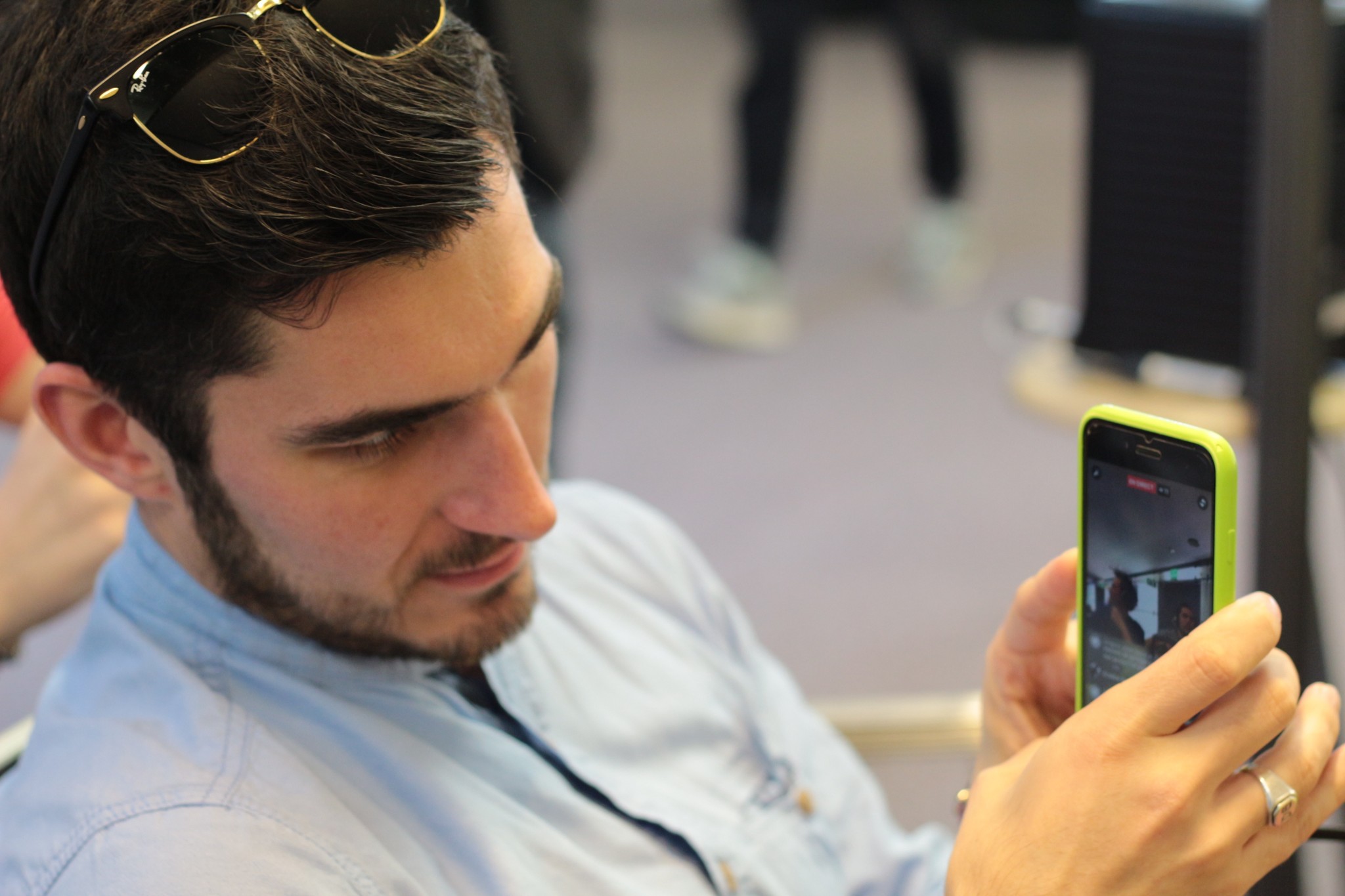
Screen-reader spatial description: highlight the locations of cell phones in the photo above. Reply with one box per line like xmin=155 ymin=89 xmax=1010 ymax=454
xmin=1073 ymin=403 xmax=1237 ymax=715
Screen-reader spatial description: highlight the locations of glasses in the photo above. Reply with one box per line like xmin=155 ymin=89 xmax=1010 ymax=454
xmin=30 ymin=0 xmax=444 ymax=322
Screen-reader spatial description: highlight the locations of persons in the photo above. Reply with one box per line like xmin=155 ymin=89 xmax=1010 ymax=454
xmin=1090 ymin=568 xmax=1145 ymax=646
xmin=1146 ymin=599 xmax=1199 ymax=658
xmin=0 ymin=0 xmax=1345 ymax=896
xmin=0 ymin=283 xmax=137 ymax=650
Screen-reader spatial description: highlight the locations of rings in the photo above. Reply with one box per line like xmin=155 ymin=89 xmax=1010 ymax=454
xmin=1236 ymin=764 xmax=1299 ymax=828
